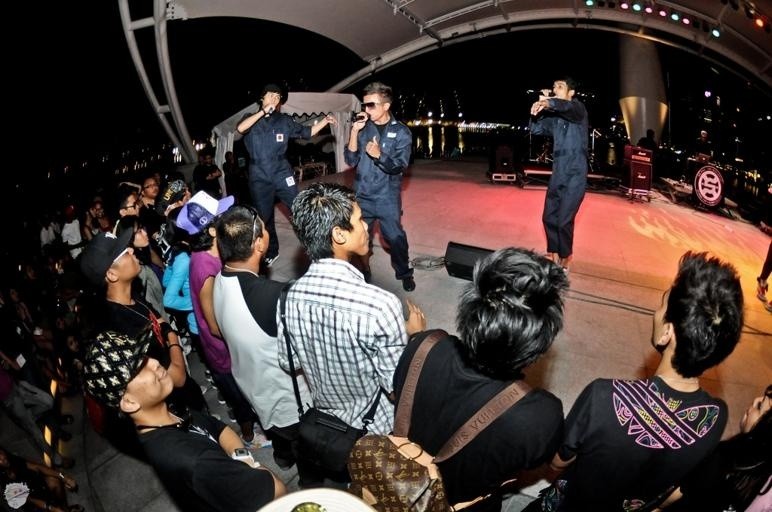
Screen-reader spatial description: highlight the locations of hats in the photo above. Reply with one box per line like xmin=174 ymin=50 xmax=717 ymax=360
xmin=80 ymin=227 xmax=133 ymax=278
xmin=175 ymin=190 xmax=235 ymax=235
xmin=254 ymin=80 xmax=288 ymax=106
xmin=78 ymin=319 xmax=153 ymax=409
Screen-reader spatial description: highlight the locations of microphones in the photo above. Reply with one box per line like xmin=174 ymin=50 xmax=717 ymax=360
xmin=346 ymin=113 xmax=370 ymax=122
xmin=527 ymin=90 xmax=555 ymax=96
xmin=269 ymin=107 xmax=272 ymax=115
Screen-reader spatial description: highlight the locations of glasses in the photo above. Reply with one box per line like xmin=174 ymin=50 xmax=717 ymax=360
xmin=122 ymin=200 xmax=138 ymax=209
xmin=236 ymin=205 xmax=257 ymax=249
xmin=361 ymin=102 xmax=382 ymax=110
xmin=144 ymin=183 xmax=158 ymax=188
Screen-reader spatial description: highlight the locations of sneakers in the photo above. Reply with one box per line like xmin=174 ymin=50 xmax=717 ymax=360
xmin=757 ymin=281 xmax=772 ymax=312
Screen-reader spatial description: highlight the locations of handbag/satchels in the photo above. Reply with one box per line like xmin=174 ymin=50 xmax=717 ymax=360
xmin=294 ymin=407 xmax=367 ymax=484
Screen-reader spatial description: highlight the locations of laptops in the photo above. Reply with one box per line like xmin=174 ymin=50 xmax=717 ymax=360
xmin=697 ymin=153 xmax=710 ymax=164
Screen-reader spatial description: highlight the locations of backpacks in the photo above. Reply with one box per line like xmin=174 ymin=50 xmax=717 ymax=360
xmin=347 ymin=432 xmax=453 ymax=512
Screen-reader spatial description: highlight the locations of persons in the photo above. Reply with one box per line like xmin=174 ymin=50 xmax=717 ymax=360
xmin=0 ymin=152 xmax=772 ymax=512
xmin=341 ymin=81 xmax=415 ymax=294
xmin=528 ymin=77 xmax=590 ymax=268
xmin=695 ymin=128 xmax=711 ymax=155
xmin=233 ymin=81 xmax=338 ymax=271
xmin=636 ymin=128 xmax=658 ymax=152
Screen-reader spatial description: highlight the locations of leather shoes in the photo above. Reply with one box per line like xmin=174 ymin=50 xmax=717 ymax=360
xmin=403 ymin=276 xmax=416 ymax=292
xmin=360 ymin=266 xmax=372 ymax=284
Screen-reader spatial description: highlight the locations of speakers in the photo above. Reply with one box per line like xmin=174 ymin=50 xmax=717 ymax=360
xmin=444 ymin=242 xmax=495 ymax=281
